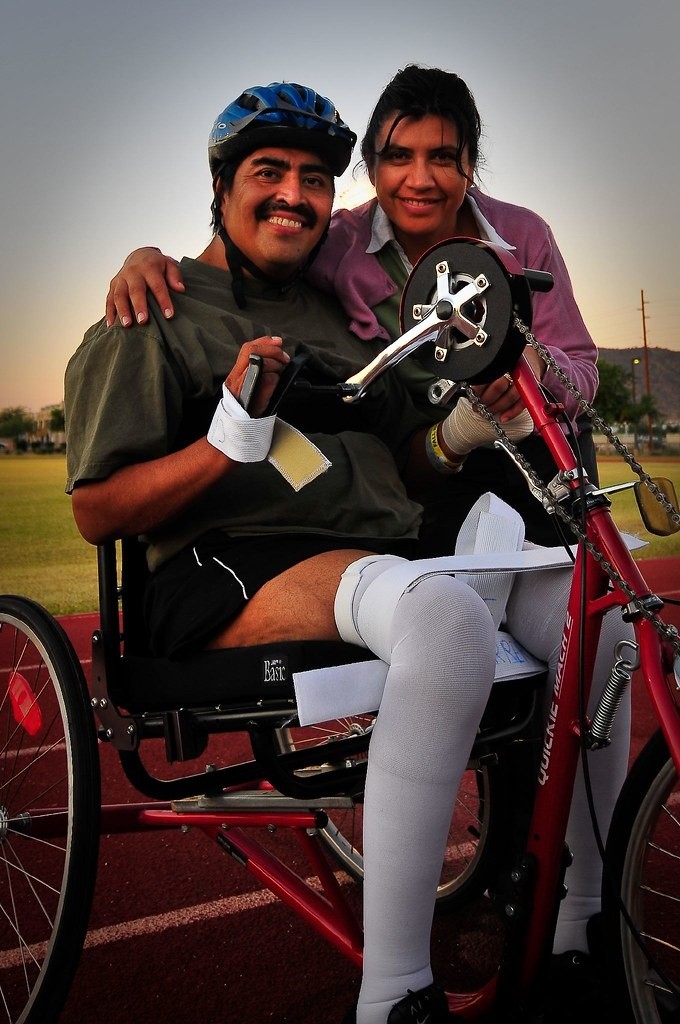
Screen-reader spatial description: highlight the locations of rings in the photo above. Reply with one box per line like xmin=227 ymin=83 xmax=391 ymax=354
xmin=504 ymin=372 xmax=515 ymax=386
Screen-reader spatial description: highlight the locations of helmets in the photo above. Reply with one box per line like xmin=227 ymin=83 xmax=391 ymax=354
xmin=208 ymin=81 xmax=357 ymax=189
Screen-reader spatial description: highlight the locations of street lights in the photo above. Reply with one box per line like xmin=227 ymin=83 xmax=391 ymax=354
xmin=630 ymin=356 xmax=640 ymax=451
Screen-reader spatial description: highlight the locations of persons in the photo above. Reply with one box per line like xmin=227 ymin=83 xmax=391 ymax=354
xmin=104 ymin=66 xmax=601 ymax=549
xmin=64 ymin=80 xmax=637 ymax=1024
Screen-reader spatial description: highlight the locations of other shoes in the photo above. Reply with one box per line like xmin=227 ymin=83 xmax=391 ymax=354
xmin=343 ymin=983 xmax=465 ymax=1023
xmin=543 ymin=936 xmax=677 ymax=1024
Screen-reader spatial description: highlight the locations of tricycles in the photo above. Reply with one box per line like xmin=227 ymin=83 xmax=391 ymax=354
xmin=0 ymin=227 xmax=680 ymax=1024
